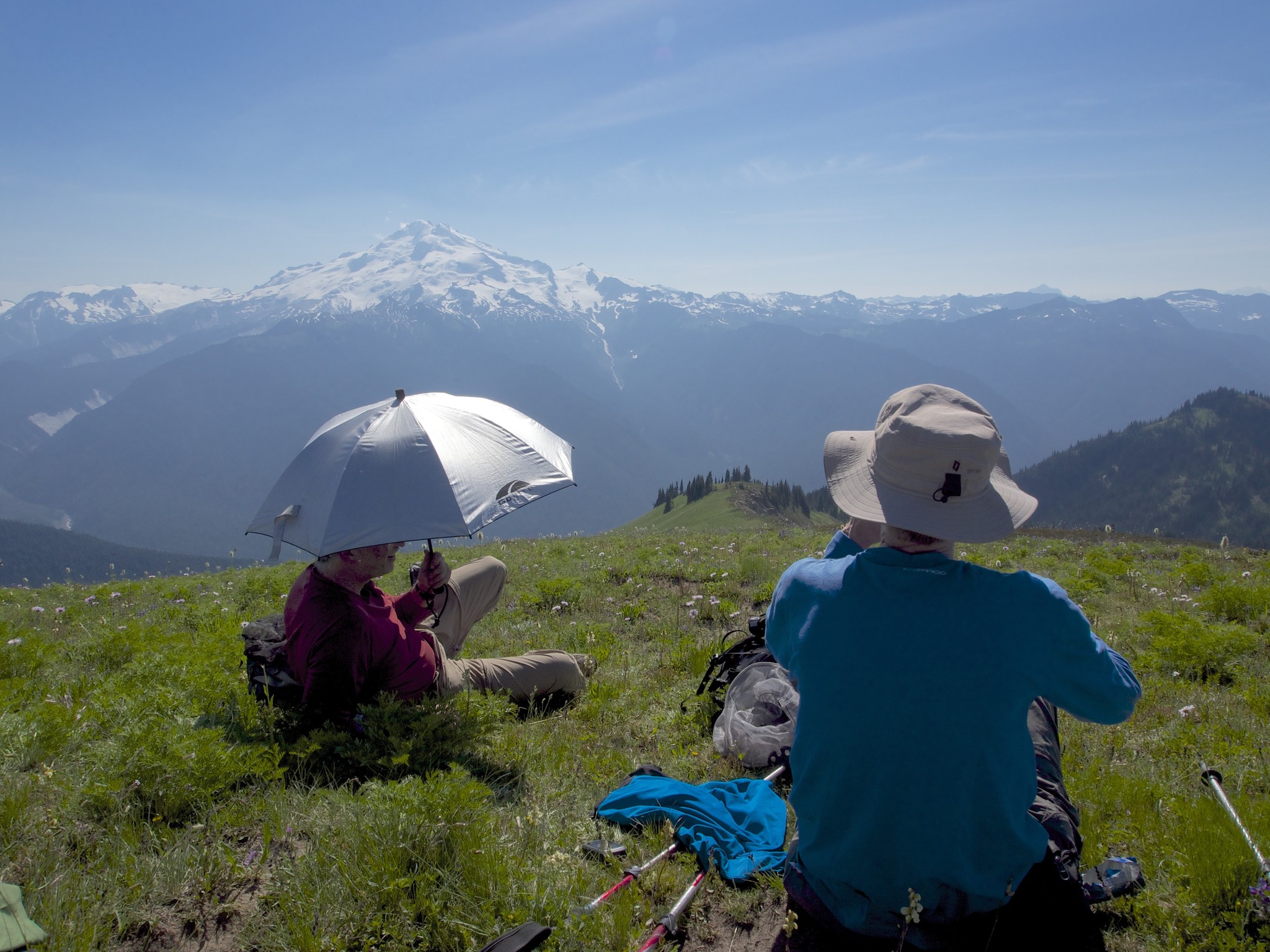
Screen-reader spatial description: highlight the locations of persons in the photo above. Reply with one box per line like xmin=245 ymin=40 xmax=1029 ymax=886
xmin=286 ymin=544 xmax=598 ymax=723
xmin=760 ymin=382 xmax=1142 ymax=951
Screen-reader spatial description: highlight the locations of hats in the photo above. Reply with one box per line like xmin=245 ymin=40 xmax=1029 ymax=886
xmin=823 ymin=385 xmax=1036 ymax=545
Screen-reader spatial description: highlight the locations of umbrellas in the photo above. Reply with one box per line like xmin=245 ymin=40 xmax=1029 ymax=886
xmin=248 ymin=388 xmax=579 ymax=592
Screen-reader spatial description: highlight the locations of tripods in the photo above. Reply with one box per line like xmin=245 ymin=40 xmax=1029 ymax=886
xmin=581 ymin=747 xmax=793 ymax=952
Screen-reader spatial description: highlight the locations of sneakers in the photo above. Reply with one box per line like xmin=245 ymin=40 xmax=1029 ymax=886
xmin=572 ymin=653 xmax=597 ymax=676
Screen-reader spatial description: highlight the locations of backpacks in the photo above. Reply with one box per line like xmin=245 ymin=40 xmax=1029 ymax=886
xmin=695 ymin=635 xmax=776 ymax=698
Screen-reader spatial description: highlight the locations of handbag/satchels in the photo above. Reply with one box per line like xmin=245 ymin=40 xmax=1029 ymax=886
xmin=245 ymin=615 xmax=297 ymax=701
